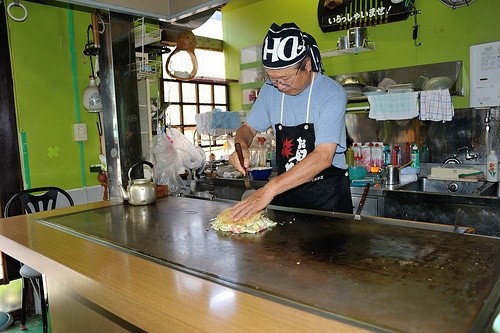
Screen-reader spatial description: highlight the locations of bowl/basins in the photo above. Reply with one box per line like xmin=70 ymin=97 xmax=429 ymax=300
xmin=340 ymin=76 xmax=387 ymax=97
xmin=377 ymin=78 xmax=396 ymax=90
xmin=247 ymin=167 xmax=273 ymax=180
xmin=215 ymin=164 xmax=237 ymax=177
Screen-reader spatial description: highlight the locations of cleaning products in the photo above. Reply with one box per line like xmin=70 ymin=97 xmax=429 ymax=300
xmin=410 ymin=143 xmax=420 ymax=168
xmin=486 ymin=150 xmax=498 ymax=183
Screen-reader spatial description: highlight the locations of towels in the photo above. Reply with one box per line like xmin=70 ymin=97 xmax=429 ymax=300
xmin=418 ymin=88 xmax=455 ymax=123
xmin=367 ymin=91 xmax=420 ymax=123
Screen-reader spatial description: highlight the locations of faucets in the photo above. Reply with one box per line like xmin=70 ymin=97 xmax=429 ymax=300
xmin=452 ymin=146 xmax=478 ymax=160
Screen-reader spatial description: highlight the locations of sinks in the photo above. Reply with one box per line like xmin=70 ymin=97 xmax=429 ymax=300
xmin=476 ymin=181 xmax=500 ymax=199
xmin=380 ymin=175 xmax=486 ymax=200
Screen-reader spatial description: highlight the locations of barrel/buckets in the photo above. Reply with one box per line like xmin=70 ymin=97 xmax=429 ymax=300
xmin=347 ymin=27 xmax=367 ymax=49
xmin=337 ymin=36 xmax=348 ymax=50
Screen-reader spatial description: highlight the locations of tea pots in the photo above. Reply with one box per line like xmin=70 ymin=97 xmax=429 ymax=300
xmin=117 ymin=160 xmax=157 ymax=206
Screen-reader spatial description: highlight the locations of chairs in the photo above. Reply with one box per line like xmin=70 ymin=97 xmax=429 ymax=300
xmin=4 ymin=187 xmax=74 ymax=333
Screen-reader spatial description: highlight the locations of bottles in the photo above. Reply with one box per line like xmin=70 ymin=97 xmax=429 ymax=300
xmin=249 ymin=134 xmax=271 ymax=167
xmin=420 ymin=143 xmax=429 ymax=163
xmin=486 ymin=150 xmax=498 ymax=182
xmin=83 ymin=75 xmax=102 ymax=112
xmin=347 ymin=140 xmax=420 ymax=174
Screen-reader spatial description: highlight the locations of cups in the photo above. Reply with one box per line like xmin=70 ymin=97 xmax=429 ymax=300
xmin=381 ymin=165 xmax=399 ymax=185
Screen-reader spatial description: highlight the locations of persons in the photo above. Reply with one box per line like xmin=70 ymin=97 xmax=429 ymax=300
xmin=228 ymin=22 xmax=353 ymax=223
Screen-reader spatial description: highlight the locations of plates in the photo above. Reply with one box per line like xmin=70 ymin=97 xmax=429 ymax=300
xmin=422 ymin=76 xmax=454 ymax=90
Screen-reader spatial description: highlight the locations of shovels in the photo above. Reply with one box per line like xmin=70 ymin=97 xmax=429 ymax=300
xmin=354 ymin=183 xmax=370 ymax=220
xmin=235 ymin=142 xmax=256 ymax=202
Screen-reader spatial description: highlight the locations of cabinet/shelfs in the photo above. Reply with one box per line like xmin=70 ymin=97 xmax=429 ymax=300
xmin=134 ymin=16 xmax=162 ymax=80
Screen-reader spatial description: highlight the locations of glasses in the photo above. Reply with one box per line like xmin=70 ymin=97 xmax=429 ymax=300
xmin=264 ymin=58 xmax=305 ymax=88
